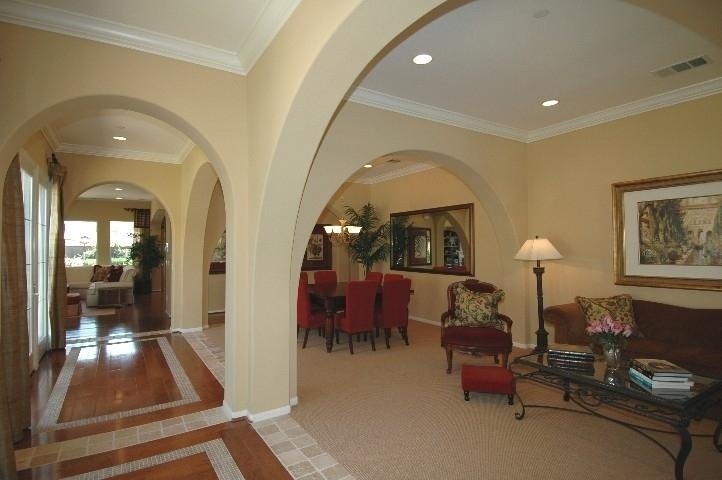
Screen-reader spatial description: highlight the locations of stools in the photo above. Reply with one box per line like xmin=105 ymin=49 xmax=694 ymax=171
xmin=462 ymin=364 xmax=515 ymax=405
xmin=67 ymin=292 xmax=82 ymax=316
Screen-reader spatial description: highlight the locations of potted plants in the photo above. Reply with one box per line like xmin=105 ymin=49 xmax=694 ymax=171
xmin=128 ymin=231 xmax=164 ymax=295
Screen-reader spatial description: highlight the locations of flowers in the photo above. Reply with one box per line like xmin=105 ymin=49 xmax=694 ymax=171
xmin=583 ymin=314 xmax=634 ymax=346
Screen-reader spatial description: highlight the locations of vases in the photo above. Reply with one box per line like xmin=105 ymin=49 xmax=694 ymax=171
xmin=602 ymin=344 xmax=621 ymax=369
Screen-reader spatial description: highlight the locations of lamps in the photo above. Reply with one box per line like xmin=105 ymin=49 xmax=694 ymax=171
xmin=324 ymin=216 xmax=364 ymax=281
xmin=511 ymin=236 xmax=564 ymax=352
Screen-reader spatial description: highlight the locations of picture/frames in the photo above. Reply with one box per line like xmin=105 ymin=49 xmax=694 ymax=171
xmin=610 ymin=168 xmax=721 ymax=293
xmin=210 ymin=230 xmax=226 ymax=274
xmin=299 ymin=223 xmax=333 ymax=272
xmin=407 ymin=226 xmax=433 ymax=267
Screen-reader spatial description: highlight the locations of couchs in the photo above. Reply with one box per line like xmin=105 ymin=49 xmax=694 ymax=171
xmin=543 ymin=295 xmax=722 ymax=374
xmin=65 ymin=265 xmax=137 ymax=306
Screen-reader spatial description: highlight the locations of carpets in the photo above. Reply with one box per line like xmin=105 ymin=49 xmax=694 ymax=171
xmin=81 ymin=299 xmax=115 ymax=315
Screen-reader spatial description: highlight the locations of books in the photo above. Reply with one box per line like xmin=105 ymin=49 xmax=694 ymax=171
xmin=547 ymin=343 xmax=594 ymax=365
xmin=549 ymin=362 xmax=596 ymax=377
xmin=627 ymin=357 xmax=696 ymax=395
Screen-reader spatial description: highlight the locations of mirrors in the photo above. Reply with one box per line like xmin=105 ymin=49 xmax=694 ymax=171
xmin=389 ymin=200 xmax=476 ymax=278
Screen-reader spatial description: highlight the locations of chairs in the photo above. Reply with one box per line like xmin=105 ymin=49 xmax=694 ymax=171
xmin=297 ymin=268 xmax=412 ymax=354
xmin=439 ymin=278 xmax=513 ymax=373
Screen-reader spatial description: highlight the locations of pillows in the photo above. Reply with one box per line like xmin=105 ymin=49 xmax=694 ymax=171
xmin=448 ymin=281 xmax=505 ymax=330
xmin=576 ymin=295 xmax=643 ymax=340
xmin=89 ymin=265 xmax=123 ymax=282
